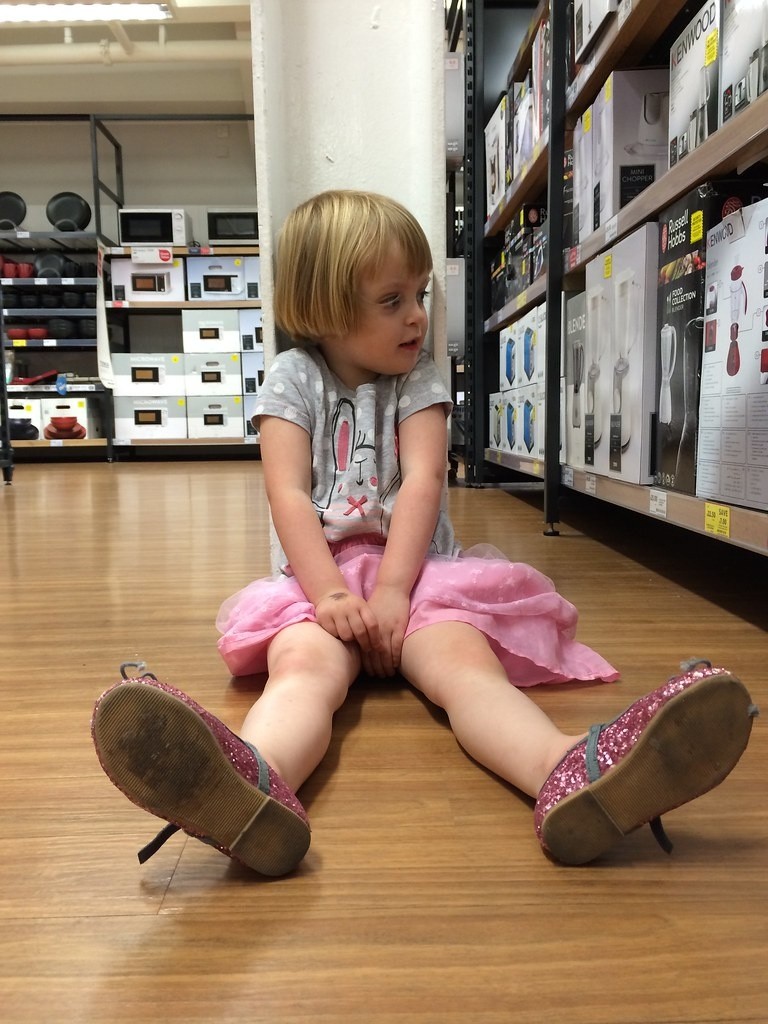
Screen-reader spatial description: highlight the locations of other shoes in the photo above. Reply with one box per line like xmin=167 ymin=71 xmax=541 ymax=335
xmin=534 ymin=655 xmax=760 ymax=866
xmin=91 ymin=663 xmax=311 ymax=877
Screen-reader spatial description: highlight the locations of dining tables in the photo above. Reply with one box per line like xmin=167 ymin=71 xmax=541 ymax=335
xmin=7 ymin=398 xmax=41 ymax=440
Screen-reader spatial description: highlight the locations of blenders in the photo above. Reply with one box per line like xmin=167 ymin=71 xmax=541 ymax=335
xmin=606 ymin=267 xmax=639 ymax=453
xmin=726 ymin=265 xmax=749 ymax=377
xmin=656 ymin=316 xmax=704 ymax=488
xmin=584 ymin=287 xmax=602 ymax=448
xmin=732 ymin=43 xmax=768 ymax=110
xmin=678 ymin=66 xmax=711 ymax=160
xmin=572 ymin=340 xmax=583 ymax=427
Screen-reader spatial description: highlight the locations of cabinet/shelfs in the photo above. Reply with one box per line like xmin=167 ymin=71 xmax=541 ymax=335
xmin=446 ymin=123 xmax=473 ymax=487
xmin=108 ymin=244 xmax=263 ymax=447
xmin=559 ymin=1 xmax=768 ymax=556
xmin=473 ymin=0 xmax=560 ymax=540
xmin=1 ymin=114 xmax=130 ymax=465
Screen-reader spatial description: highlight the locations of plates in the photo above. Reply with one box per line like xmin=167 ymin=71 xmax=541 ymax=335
xmin=45 ymin=192 xmax=93 ymax=232
xmin=0 ymin=190 xmax=26 ymax=231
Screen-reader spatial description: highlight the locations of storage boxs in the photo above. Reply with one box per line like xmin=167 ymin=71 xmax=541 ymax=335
xmin=566 ymin=291 xmax=585 ymax=470
xmin=564 ymin=69 xmax=673 ymax=250
xmin=668 ymin=1 xmax=767 ymax=169
xmin=499 ymin=303 xmax=549 ymax=393
xmin=447 ymin=258 xmax=466 ymax=356
xmin=110 ymin=259 xmax=186 ymax=301
xmin=583 ymin=222 xmax=655 ymax=487
xmin=239 ymin=309 xmax=263 ymax=352
xmin=573 ymin=1 xmax=620 ymax=64
xmin=110 ymin=352 xmax=263 ymax=438
xmin=40 ymin=396 xmax=103 ymax=439
xmin=185 ymin=256 xmax=261 ymax=300
xmin=179 ymin=309 xmax=240 ymax=352
xmin=655 ymin=180 xmax=767 ymax=514
xmin=453 ymin=404 xmax=469 ymax=456
xmin=487 ymin=378 xmax=565 ymax=465
xmin=484 ymin=2 xmax=549 ymax=311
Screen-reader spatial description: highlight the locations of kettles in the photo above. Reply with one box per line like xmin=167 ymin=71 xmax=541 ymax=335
xmin=638 ymin=90 xmax=672 ymax=147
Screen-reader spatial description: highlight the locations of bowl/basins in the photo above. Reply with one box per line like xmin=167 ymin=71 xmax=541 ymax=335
xmin=50 ymin=417 xmax=77 ymax=429
xmin=0 ymin=252 xmax=97 ymax=340
xmin=8 ymin=418 xmax=31 ymax=430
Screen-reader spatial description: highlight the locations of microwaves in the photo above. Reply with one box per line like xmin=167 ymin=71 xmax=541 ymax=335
xmin=198 ymin=323 xmax=225 ymax=342
xmin=205 ymin=206 xmax=260 ymax=246
xmin=203 ymin=272 xmax=242 ymax=295
xmin=116 ymin=207 xmax=195 ymax=247
xmin=203 ymin=408 xmax=229 ymax=426
xmin=127 ymin=358 xmax=166 ymax=385
xmin=200 ymin=368 xmax=226 ymax=383
xmin=131 ymin=408 xmax=168 ymax=427
xmin=130 ymin=272 xmax=171 ymax=294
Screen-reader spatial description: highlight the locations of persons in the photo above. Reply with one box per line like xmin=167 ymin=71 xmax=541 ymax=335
xmin=89 ymin=189 xmax=757 ymax=883
